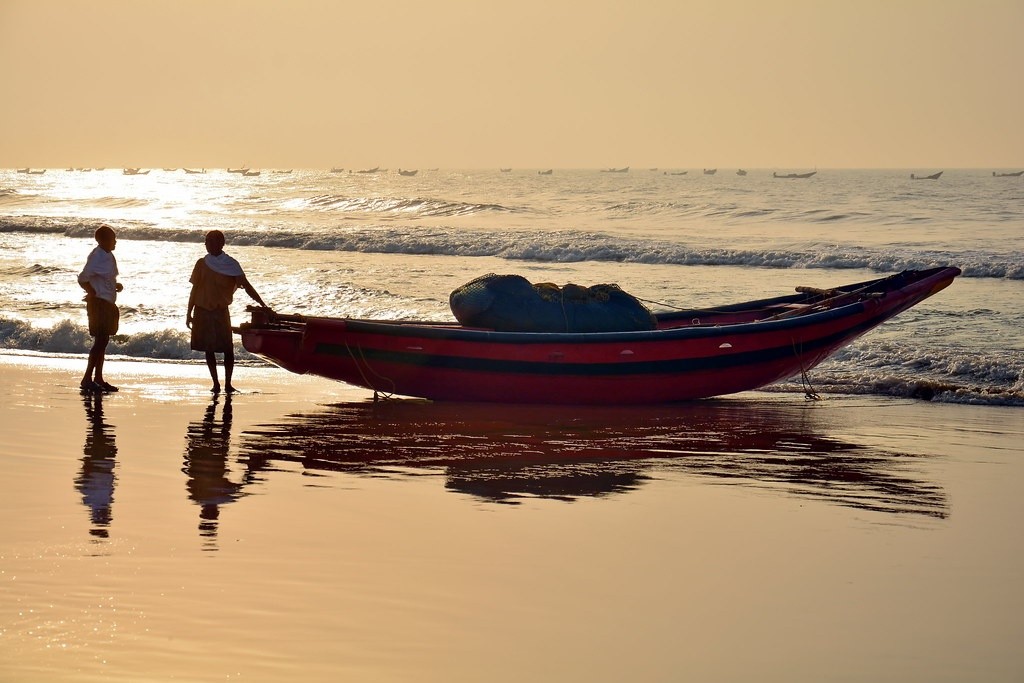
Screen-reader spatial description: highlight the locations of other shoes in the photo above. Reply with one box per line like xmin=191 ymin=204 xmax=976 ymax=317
xmin=80 ymin=378 xmax=101 ymax=392
xmin=99 ymin=382 xmax=118 ymax=392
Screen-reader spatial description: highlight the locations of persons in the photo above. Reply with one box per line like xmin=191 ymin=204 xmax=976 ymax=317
xmin=186 ymin=230 xmax=270 ymax=393
xmin=76 ymin=393 xmax=117 ymax=539
xmin=78 ymin=226 xmax=122 ymax=393
xmin=184 ymin=394 xmax=254 ymax=541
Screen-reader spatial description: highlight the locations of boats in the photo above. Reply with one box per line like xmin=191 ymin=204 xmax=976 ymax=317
xmin=231 ymin=265 xmax=962 ymax=408
